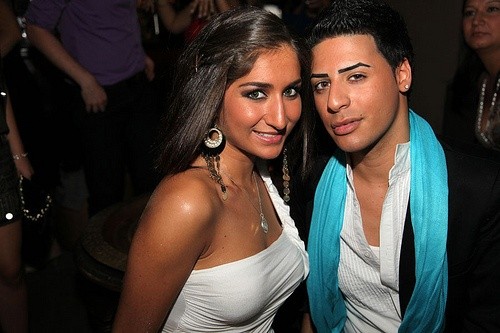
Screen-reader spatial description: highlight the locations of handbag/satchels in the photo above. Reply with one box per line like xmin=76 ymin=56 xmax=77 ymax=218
xmin=19 ymin=172 xmax=52 ymax=221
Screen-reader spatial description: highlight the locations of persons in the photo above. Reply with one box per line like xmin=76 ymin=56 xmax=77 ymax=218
xmin=443 ymin=0 xmax=500 ymax=162
xmin=113 ymin=7 xmax=310 ymax=333
xmin=288 ymin=0 xmax=500 ymax=333
xmin=0 ymin=0 xmax=242 ymax=333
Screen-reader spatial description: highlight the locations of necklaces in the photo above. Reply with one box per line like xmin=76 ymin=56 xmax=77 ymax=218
xmin=228 ymin=171 xmax=268 ymax=234
xmin=478 ymin=75 xmax=500 ymax=143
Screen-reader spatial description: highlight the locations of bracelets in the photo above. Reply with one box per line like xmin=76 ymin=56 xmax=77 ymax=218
xmin=12 ymin=152 xmax=28 ymax=160
